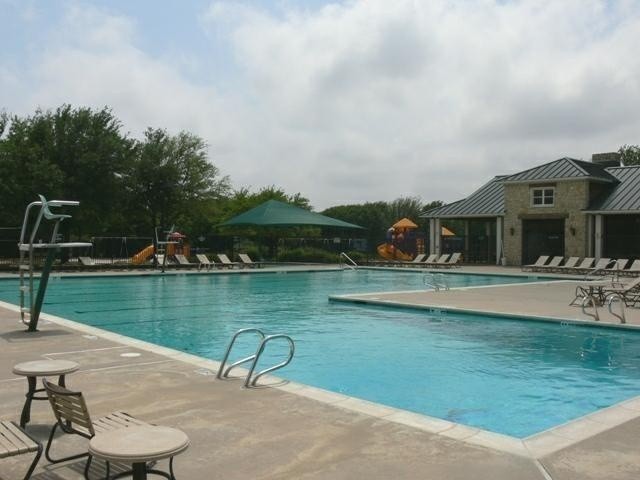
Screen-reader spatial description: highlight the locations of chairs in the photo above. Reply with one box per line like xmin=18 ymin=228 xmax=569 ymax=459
xmin=0 ymin=419 xmax=43 ymax=479
xmin=520 ymin=255 xmax=640 ymax=309
xmin=38 ymin=192 xmax=72 ymax=243
xmin=368 ymin=252 xmax=462 ymax=268
xmin=153 ymin=253 xmax=266 ymax=271
xmin=78 ymin=256 xmax=100 ymax=272
xmin=41 ymin=377 xmax=161 ymax=480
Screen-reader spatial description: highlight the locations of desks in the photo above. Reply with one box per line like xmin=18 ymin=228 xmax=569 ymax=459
xmin=12 ymin=359 xmax=80 ymax=430
xmin=83 ymin=425 xmax=189 ymax=480
